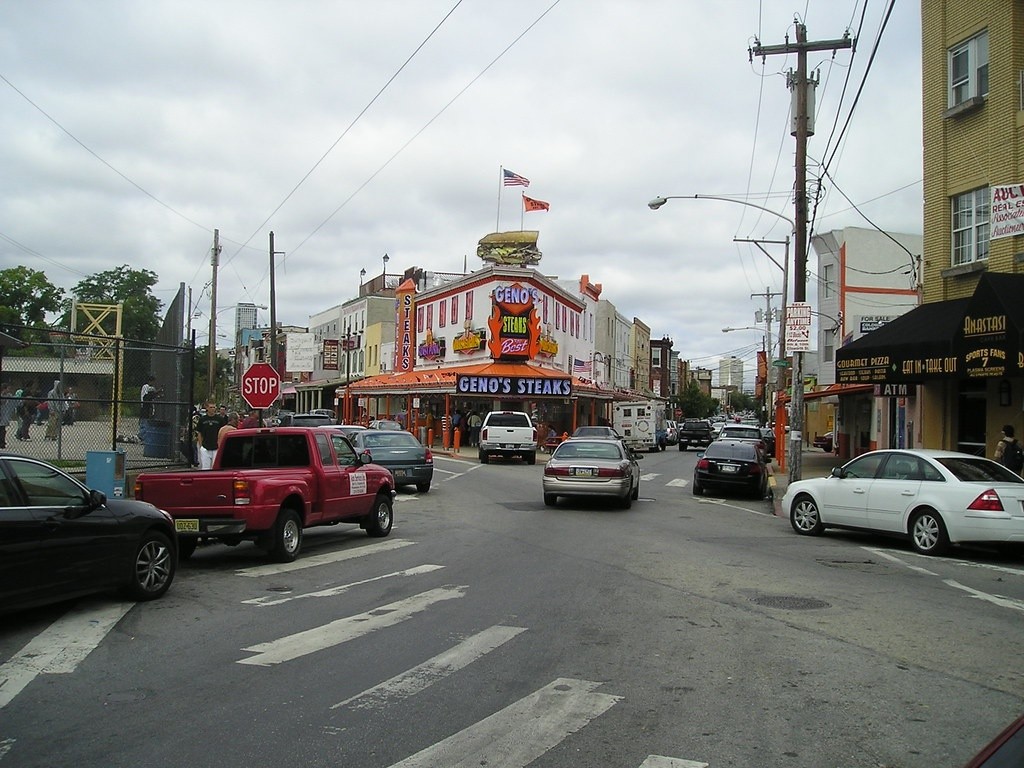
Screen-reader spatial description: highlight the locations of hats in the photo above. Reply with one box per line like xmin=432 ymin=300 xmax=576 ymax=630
xmin=148 ymin=387 xmax=155 ymax=391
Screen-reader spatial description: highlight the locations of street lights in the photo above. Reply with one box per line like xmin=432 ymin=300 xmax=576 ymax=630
xmin=208 ymin=304 xmax=268 ymax=398
xmin=647 ymin=194 xmax=808 ymax=486
xmin=722 ymin=326 xmax=786 ymax=399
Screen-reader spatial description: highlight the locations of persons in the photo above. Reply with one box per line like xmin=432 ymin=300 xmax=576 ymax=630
xmin=15 ymin=383 xmax=43 ymax=441
xmin=217 ymin=411 xmax=238 ymax=446
xmin=426 ymin=410 xmax=434 ymax=429
xmin=218 ymin=406 xmax=228 ymax=421
xmin=0 ymin=382 xmax=21 ymax=449
xmin=454 ymin=407 xmax=482 ymax=447
xmin=195 ymin=400 xmax=226 ymax=469
xmin=137 ymin=376 xmax=165 ymax=440
xmin=237 ymin=410 xmax=258 ymax=429
xmin=186 ymin=406 xmax=201 ymax=467
xmin=546 ymin=426 xmax=556 ymax=442
xmin=45 ymin=381 xmax=60 ymax=441
xmin=63 ymin=386 xmax=80 ymax=426
xmin=993 ymin=424 xmax=1024 ymax=477
xmin=442 ymin=411 xmax=453 ymax=451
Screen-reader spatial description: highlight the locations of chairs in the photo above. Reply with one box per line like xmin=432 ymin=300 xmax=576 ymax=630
xmin=559 ymin=446 xmax=576 ymax=455
xmin=894 ymin=462 xmax=912 ymax=478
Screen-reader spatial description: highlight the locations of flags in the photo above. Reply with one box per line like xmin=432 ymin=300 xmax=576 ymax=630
xmin=523 ymin=194 xmax=550 ymax=212
xmin=504 ymin=169 xmax=530 ymax=187
xmin=573 ymin=359 xmax=592 ymax=372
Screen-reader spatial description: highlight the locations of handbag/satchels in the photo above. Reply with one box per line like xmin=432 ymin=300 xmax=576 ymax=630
xmin=467 ymin=425 xmax=471 ymax=432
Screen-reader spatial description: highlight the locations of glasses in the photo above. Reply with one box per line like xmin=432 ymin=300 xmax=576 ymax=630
xmin=210 ymin=407 xmax=216 ymax=409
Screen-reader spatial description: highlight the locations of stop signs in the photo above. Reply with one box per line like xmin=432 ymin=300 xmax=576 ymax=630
xmin=240 ymin=362 xmax=281 ymax=410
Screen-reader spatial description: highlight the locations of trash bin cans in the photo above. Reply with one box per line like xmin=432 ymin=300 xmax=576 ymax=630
xmin=84 ymin=447 xmax=127 ymax=506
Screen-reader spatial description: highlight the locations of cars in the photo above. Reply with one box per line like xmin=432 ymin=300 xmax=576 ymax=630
xmin=565 ymin=425 xmax=629 ymax=459
xmin=0 ymin=451 xmax=181 ymax=620
xmin=812 ymin=432 xmax=834 ymax=453
xmin=332 ymin=428 xmax=434 ymax=493
xmin=778 ymin=448 xmax=1023 ymax=558
xmin=543 ymin=439 xmax=644 ymax=509
xmin=665 ymin=411 xmax=777 ymax=463
xmin=262 ymin=407 xmax=406 ymax=457
xmin=692 ymin=440 xmax=773 ymax=500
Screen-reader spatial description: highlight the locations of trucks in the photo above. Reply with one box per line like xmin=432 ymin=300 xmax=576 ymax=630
xmin=612 ymin=399 xmax=669 ymax=452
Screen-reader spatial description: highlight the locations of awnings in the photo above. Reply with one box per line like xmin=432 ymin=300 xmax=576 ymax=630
xmin=835 ymin=272 xmax=1024 ymax=384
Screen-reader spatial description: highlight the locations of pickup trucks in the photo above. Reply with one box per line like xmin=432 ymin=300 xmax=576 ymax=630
xmin=133 ymin=423 xmax=396 ymax=564
xmin=676 ymin=420 xmax=715 ymax=451
xmin=474 ymin=410 xmax=538 ymax=465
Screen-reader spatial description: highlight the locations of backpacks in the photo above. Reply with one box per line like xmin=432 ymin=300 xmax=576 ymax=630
xmin=1000 ymin=439 xmax=1022 ymax=472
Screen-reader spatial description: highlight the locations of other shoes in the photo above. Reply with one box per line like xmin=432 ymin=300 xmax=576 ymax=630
xmin=45 ymin=436 xmax=59 ymax=441
xmin=21 ymin=438 xmax=32 ymax=442
xmin=194 ymin=462 xmax=200 ymax=467
